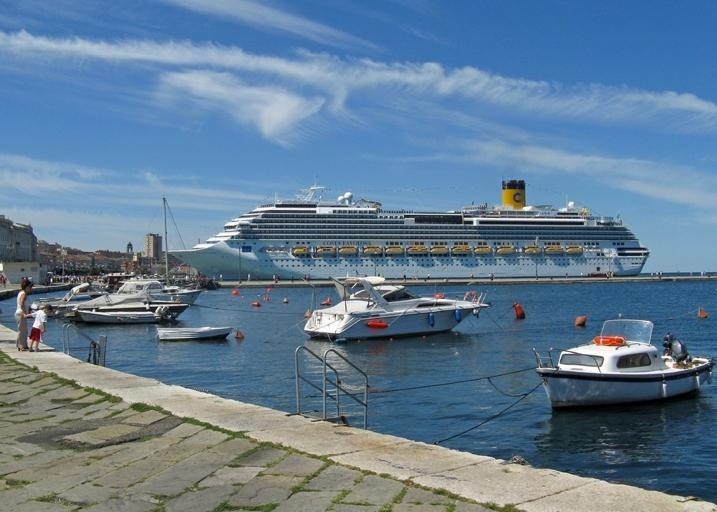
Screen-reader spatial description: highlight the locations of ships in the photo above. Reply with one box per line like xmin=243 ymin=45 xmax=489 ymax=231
xmin=164 ymin=179 xmax=652 ymax=280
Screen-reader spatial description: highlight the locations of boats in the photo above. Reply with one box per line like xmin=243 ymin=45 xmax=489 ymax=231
xmin=28 ymin=196 xmax=205 ymax=325
xmin=532 ymin=319 xmax=712 ymax=408
xmin=303 ymin=276 xmax=492 ymax=342
xmin=156 ymin=326 xmax=234 ymax=341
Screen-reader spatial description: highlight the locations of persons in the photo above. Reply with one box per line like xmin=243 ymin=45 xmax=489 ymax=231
xmin=24 ymin=304 xmax=53 ymax=352
xmin=14 ymin=279 xmax=34 ymax=351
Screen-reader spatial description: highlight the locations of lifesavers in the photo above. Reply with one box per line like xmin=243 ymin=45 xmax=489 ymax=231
xmin=593 ymin=336 xmax=625 ymax=346
